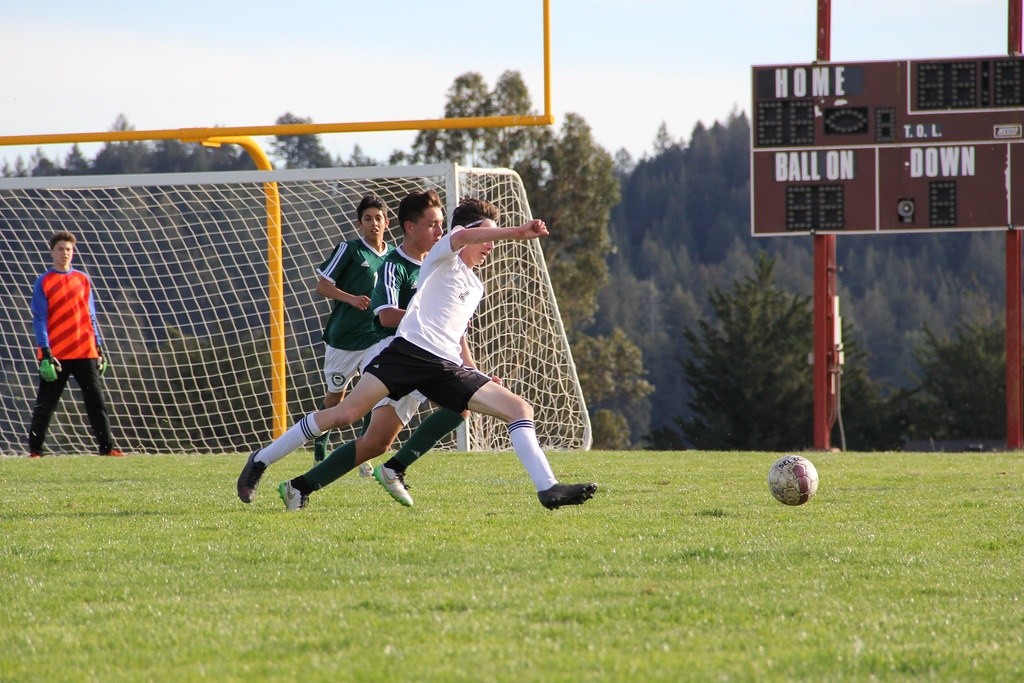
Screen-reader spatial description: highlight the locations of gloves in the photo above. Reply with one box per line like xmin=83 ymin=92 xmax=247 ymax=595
xmin=95 ymin=346 xmax=107 ymax=378
xmin=36 ymin=348 xmax=62 ymax=382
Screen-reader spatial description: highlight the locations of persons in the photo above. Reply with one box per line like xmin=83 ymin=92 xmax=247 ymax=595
xmin=29 ymin=232 xmax=124 ymax=457
xmin=276 ymin=192 xmax=470 ymax=509
xmin=236 ymin=197 xmax=598 ymax=510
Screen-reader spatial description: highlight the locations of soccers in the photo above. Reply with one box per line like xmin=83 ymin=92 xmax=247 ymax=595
xmin=768 ymin=455 xmax=820 ymax=506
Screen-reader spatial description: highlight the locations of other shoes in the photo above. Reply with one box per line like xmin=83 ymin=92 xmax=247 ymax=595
xmin=359 ymin=462 xmax=373 ymax=476
xmin=100 ymin=448 xmax=129 ymax=457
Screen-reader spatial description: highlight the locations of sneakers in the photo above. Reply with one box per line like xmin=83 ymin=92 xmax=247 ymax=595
xmin=537 ymin=483 xmax=598 ymax=510
xmin=372 ymin=464 xmax=414 ymax=508
xmin=237 ymin=449 xmax=268 ymax=503
xmin=277 ymin=480 xmax=306 ymax=512
